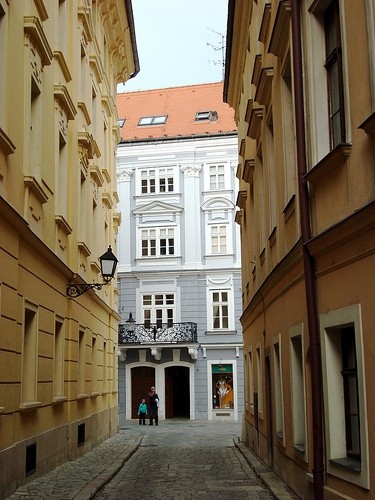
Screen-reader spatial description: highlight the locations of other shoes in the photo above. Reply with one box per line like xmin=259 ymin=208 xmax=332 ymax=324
xmin=139 ymin=423 xmax=142 ymax=425
xmin=155 ymin=422 xmax=158 ymax=426
xmin=149 ymin=423 xmax=154 ymax=426
xmin=143 ymin=423 xmax=147 ymax=425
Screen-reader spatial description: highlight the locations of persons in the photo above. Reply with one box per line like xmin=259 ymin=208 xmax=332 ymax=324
xmin=216 ymin=373 xmax=227 ymax=408
xmin=147 ymin=387 xmax=160 ymax=426
xmin=138 ymin=399 xmax=148 ymax=425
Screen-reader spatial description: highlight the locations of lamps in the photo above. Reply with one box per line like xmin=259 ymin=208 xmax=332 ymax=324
xmin=119 ymin=312 xmax=136 ymax=341
xmin=66 ymin=245 xmax=119 ymax=297
